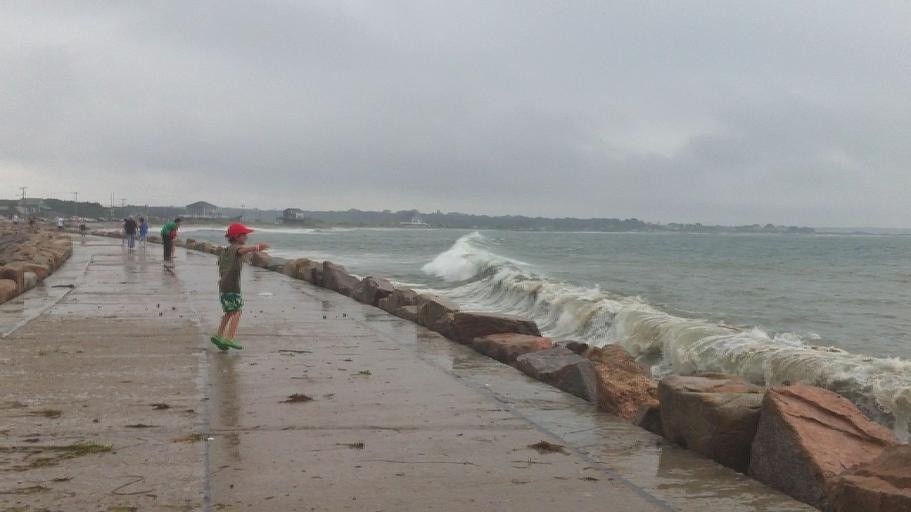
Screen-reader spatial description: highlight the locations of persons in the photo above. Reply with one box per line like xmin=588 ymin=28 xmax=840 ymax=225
xmin=139 ymin=216 xmax=149 ymax=249
xmin=160 ymin=217 xmax=180 ymax=267
xmin=58 ymin=216 xmax=65 ymax=232
xmin=127 ymin=215 xmax=136 ymax=249
xmin=210 ymin=222 xmax=276 ymax=351
xmin=79 ymin=222 xmax=86 ymax=237
xmin=121 ymin=219 xmax=127 ymax=246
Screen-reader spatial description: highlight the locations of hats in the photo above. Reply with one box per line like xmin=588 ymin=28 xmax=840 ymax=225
xmin=225 ymin=223 xmax=254 ymax=238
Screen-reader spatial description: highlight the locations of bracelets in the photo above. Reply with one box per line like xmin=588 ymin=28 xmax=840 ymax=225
xmin=256 ymin=244 xmax=260 ymax=252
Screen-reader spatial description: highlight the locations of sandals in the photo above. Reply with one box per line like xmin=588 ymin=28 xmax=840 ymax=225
xmin=211 ymin=334 xmax=243 ymax=350
